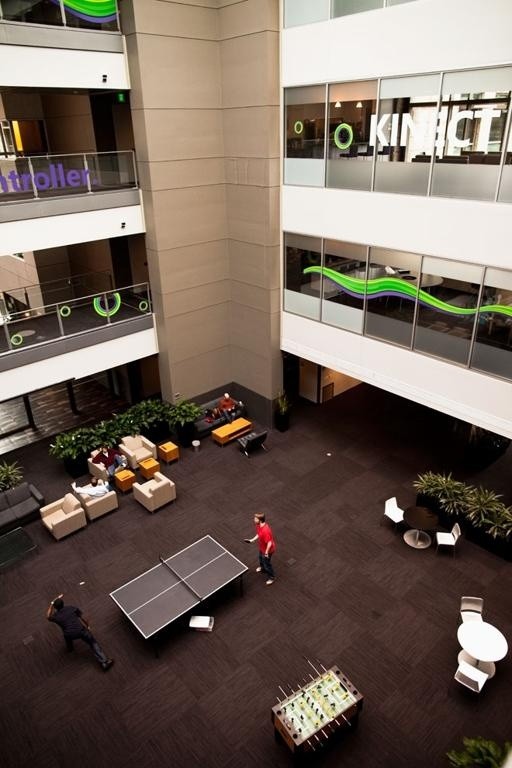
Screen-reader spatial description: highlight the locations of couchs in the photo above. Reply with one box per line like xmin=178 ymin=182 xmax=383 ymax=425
xmin=1 ymin=481 xmax=46 ymax=530
xmin=192 ymin=395 xmax=245 ymax=439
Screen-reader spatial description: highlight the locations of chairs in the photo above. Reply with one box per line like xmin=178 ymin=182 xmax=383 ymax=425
xmin=380 ymin=496 xmax=405 ymax=535
xmin=339 ymin=143 xmax=512 ymax=165
xmin=237 ymin=430 xmax=268 ymax=456
xmin=453 ymin=660 xmax=489 ymax=712
xmin=71 ymin=478 xmax=120 ymax=521
xmin=88 ymin=446 xmax=128 ymax=480
xmin=119 ymin=434 xmax=158 ymax=470
xmin=39 ymin=493 xmax=87 ymax=542
xmin=432 ymin=522 xmax=461 ymax=560
xmin=457 ymin=596 xmax=484 ymax=623
xmin=132 ymin=471 xmax=177 ymax=514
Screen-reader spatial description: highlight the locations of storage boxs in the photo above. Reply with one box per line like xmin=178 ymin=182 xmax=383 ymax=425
xmin=189 ymin=614 xmax=216 ymax=633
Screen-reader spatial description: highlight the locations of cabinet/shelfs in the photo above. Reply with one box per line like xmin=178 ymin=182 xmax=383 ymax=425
xmin=310 ymin=259 xmax=361 ymax=283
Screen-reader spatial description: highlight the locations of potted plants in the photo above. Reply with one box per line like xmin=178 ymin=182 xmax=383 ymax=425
xmin=49 ymin=398 xmax=170 ymax=479
xmin=169 ymin=401 xmax=204 ymax=449
xmin=411 ymin=470 xmax=511 ymax=564
xmin=274 ymin=389 xmax=290 ymax=433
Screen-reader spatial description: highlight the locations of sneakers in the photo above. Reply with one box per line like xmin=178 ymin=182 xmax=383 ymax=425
xmin=100 ymin=658 xmax=115 ymax=674
xmin=255 ymin=566 xmax=262 ymax=573
xmin=265 ymin=576 xmax=277 ymax=586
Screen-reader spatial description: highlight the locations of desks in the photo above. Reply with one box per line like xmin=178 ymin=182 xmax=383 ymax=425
xmin=211 ymin=416 xmax=253 ymax=448
xmin=402 ymin=506 xmax=439 ymax=549
xmin=456 ymin=622 xmax=509 ymax=680
xmin=114 ymin=468 xmax=137 ymax=493
xmin=109 ymin=532 xmax=250 ymax=659
xmin=0 ymin=526 xmax=39 ymax=570
xmin=383 ymin=267 xmax=445 ymax=312
xmin=159 ymin=441 xmax=180 ymax=465
xmin=138 ymin=457 xmax=161 ymax=480
